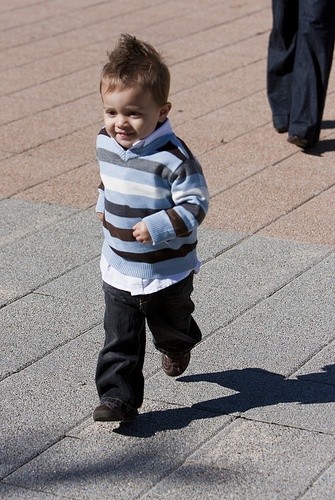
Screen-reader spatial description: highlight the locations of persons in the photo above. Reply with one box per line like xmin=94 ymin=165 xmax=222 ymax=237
xmin=90 ymin=32 xmax=210 ymax=423
xmin=265 ymin=1 xmax=335 ymax=155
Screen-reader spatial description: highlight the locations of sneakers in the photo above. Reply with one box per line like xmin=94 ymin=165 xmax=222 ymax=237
xmin=93 ymin=405 xmax=139 ymax=421
xmin=161 ymin=352 xmax=190 ymax=376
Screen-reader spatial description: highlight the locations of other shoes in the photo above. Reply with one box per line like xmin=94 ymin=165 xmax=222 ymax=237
xmin=286 ymin=134 xmax=317 ymax=148
